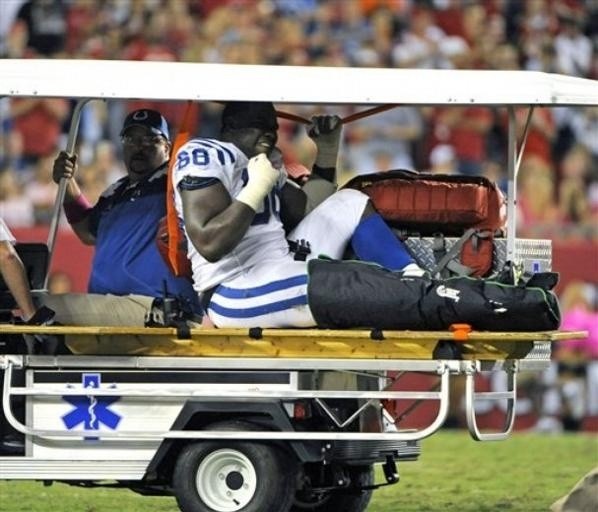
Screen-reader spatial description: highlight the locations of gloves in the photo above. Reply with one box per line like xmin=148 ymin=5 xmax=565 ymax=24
xmin=234 ymin=152 xmax=282 ymax=214
xmin=304 ymin=115 xmax=344 ymax=170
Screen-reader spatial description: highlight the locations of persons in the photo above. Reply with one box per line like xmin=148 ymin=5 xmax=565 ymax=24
xmin=1 ymin=0 xmax=598 ymax=436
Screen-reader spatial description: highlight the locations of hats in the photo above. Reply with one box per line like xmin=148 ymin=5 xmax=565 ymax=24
xmin=117 ymin=107 xmax=171 ymax=142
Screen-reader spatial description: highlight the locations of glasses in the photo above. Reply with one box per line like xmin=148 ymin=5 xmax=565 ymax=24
xmin=119 ymin=134 xmax=161 ymax=148
